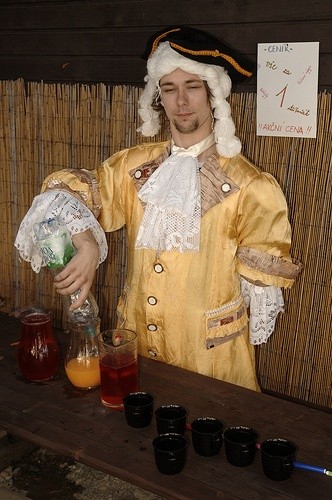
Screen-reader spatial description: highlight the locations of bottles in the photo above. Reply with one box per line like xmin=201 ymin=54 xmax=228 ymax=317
xmin=17 ymin=307 xmax=60 ymax=383
xmin=64 ymin=315 xmax=105 ymax=391
xmin=31 ymin=214 xmax=100 ymax=342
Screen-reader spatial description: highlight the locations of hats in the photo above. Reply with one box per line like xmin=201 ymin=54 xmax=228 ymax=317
xmin=142 ymin=26 xmax=253 ymax=81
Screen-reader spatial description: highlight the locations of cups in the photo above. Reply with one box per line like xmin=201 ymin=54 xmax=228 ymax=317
xmin=224 ymin=426 xmax=258 ymax=467
xmin=155 ymin=404 xmax=188 ymax=435
xmin=123 ymin=391 xmax=153 ymax=429
xmin=96 ymin=329 xmax=139 ymax=408
xmin=190 ymin=416 xmax=225 ymax=458
xmin=261 ymin=438 xmax=297 ymax=481
xmin=152 ymin=433 xmax=189 ymax=475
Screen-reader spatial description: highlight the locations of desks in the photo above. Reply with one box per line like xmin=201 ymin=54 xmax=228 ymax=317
xmin=0 ymin=311 xmax=332 ymax=500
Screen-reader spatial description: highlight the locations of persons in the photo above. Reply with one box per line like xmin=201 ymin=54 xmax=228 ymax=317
xmin=14 ymin=26 xmax=303 ymax=395
